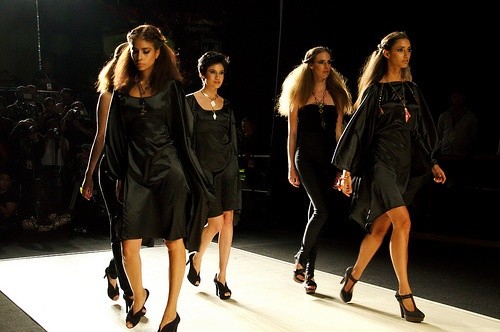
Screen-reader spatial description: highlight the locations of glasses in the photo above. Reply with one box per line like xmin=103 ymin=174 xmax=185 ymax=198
xmin=314 ymin=60 xmax=333 ymax=65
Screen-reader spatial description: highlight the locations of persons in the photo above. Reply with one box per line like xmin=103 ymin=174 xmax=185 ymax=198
xmin=105 ymin=24 xmax=216 ymax=332
xmin=236 ymin=116 xmax=260 ymax=183
xmin=437 ymin=89 xmax=477 ymax=230
xmin=0 ymin=168 xmax=18 ymax=242
xmin=275 ymin=47 xmax=353 ymax=294
xmin=331 ymin=31 xmax=447 ymax=322
xmin=0 ymin=84 xmax=116 ymax=234
xmin=80 ymin=43 xmax=146 ymax=316
xmin=186 ymin=52 xmax=242 ymax=300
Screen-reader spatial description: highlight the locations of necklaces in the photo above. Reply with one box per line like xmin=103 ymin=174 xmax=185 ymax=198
xmin=133 ymin=79 xmax=152 ymax=115
xmin=386 ymin=80 xmax=411 ymax=122
xmin=313 ymin=91 xmax=327 ymax=130
xmin=201 ymin=89 xmax=218 ymax=120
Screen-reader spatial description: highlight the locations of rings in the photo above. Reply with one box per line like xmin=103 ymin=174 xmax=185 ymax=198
xmin=80 ymin=187 xmax=82 ymax=193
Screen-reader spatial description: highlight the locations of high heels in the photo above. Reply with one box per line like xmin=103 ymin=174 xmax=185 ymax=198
xmin=104 ymin=267 xmax=119 ymax=301
xmin=340 ymin=265 xmax=357 ymax=302
xmin=304 ymin=273 xmax=317 ymax=293
xmin=125 ymin=287 xmax=150 ymax=329
xmin=213 ymin=272 xmax=231 ymax=299
xmin=293 ymin=256 xmax=306 ymax=284
xmin=185 ymin=253 xmax=201 ymax=286
xmin=395 ymin=289 xmax=426 ymax=322
xmin=158 ymin=311 xmax=180 ymax=332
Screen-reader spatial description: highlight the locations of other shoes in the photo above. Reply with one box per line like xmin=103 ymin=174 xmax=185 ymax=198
xmin=122 ymin=294 xmax=147 ymax=316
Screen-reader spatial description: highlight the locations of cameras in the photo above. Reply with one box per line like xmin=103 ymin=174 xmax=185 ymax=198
xmin=48 ymin=128 xmax=60 ymax=137
xmin=72 ymin=109 xmax=84 ymax=119
